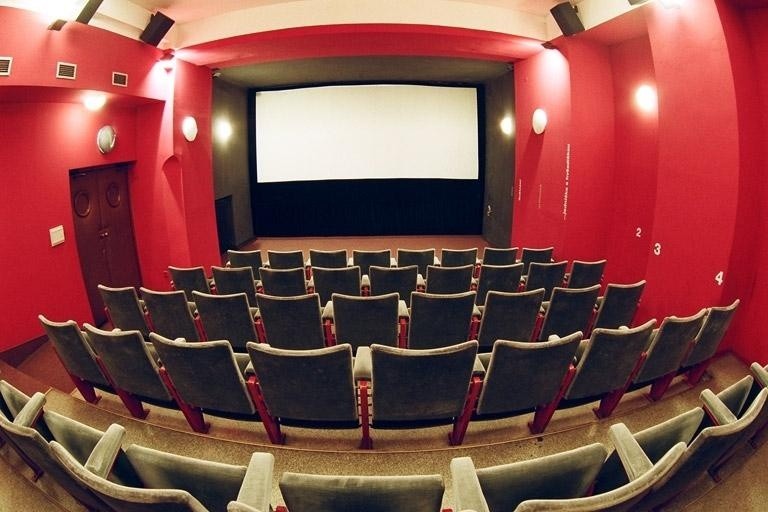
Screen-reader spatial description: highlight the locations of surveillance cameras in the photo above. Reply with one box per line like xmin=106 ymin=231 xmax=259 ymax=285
xmin=213 ymin=72 xmax=222 ymax=80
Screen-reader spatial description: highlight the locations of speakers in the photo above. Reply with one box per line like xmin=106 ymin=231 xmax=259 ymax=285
xmin=550 ymin=2 xmax=584 ymax=36
xmin=140 ymin=11 xmax=175 ymax=47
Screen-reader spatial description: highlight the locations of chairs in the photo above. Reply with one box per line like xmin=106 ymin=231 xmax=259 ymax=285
xmin=2 ymin=247 xmax=767 ymax=510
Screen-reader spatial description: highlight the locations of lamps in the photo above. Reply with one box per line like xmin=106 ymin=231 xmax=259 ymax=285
xmin=183 ymin=117 xmax=197 ymax=141
xmin=533 ymin=110 xmax=547 ymax=136
